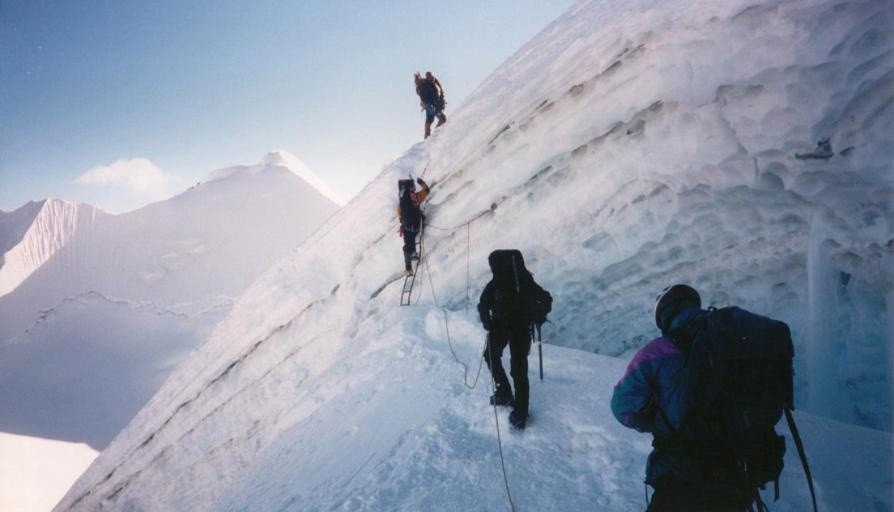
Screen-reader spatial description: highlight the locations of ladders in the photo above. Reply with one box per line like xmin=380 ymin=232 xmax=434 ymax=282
xmin=400 ymin=219 xmax=423 ymax=306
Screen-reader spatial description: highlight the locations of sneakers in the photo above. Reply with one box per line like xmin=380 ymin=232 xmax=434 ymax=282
xmin=404 ymin=270 xmax=414 ymax=276
xmin=508 ymin=408 xmax=526 ymax=431
xmin=490 ymin=393 xmax=513 ymax=405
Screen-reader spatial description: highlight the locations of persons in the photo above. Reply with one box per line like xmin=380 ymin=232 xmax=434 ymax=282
xmin=396 ymin=177 xmax=429 ymax=276
xmin=609 ymin=282 xmax=758 ymax=512
xmin=475 ymin=248 xmax=532 ymax=435
xmin=415 ymin=70 xmax=446 ymax=140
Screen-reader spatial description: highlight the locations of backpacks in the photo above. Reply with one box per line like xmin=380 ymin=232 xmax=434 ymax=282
xmin=680 ymin=307 xmax=795 ymax=487
xmin=489 ymin=249 xmax=553 ymax=327
xmin=414 ymin=73 xmax=427 ymax=110
xmin=398 ymin=178 xmax=424 ymax=235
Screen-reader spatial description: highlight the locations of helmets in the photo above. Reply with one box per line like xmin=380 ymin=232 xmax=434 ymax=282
xmin=655 ymin=283 xmax=701 ymax=329
